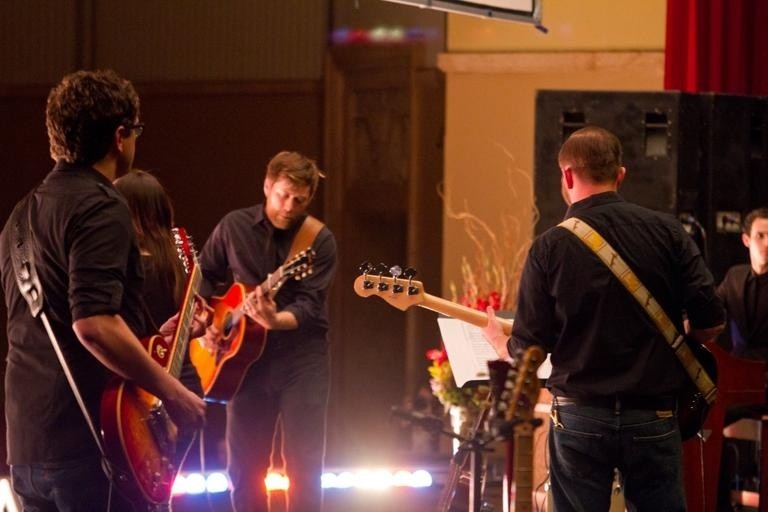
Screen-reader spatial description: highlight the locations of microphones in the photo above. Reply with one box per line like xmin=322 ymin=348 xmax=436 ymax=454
xmin=391 ymin=404 xmax=444 ymax=433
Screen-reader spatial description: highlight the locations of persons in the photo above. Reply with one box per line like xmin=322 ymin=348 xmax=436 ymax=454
xmin=714 ymin=206 xmax=768 ymax=362
xmin=195 ymin=149 xmax=338 ymax=512
xmin=110 ymin=169 xmax=212 ymax=512
xmin=1 ymin=69 xmax=216 ymax=512
xmin=480 ymin=126 xmax=729 ymax=512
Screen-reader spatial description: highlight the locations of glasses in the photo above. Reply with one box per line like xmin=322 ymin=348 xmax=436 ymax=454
xmin=123 ymin=122 xmax=145 ymax=138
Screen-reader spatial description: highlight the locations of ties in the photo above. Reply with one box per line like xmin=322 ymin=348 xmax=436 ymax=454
xmin=744 ymin=274 xmax=762 ymax=317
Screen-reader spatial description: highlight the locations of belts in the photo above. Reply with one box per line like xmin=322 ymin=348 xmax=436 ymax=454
xmin=550 ymin=395 xmax=677 ymax=414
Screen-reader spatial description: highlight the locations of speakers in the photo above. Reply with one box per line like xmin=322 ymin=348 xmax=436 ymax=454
xmin=699 ymin=93 xmax=768 ymax=229
xmin=534 ymin=89 xmax=695 ymax=234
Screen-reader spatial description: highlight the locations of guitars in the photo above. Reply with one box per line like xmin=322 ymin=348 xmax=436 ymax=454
xmin=99 ymin=226 xmax=204 ymax=506
xmin=189 ymin=247 xmax=318 ymax=403
xmin=353 ymin=261 xmax=718 ymax=440
